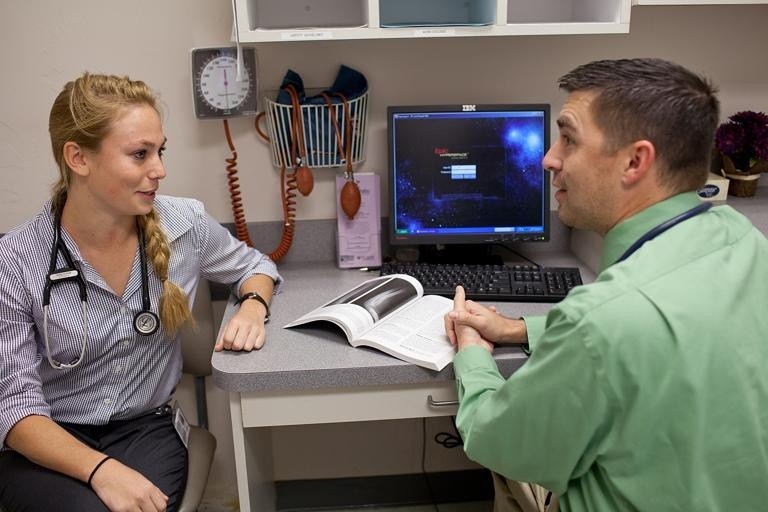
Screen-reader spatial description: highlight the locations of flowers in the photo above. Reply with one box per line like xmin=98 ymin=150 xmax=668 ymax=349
xmin=715 ymin=111 xmax=768 ymax=167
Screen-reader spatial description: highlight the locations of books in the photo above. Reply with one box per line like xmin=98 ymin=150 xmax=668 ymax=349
xmin=282 ymin=273 xmax=457 ymax=371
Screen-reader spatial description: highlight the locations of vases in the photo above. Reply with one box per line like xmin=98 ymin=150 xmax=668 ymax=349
xmin=717 ymin=152 xmax=767 ymax=198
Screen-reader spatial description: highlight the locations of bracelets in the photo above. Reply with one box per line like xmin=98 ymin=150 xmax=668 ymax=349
xmin=232 ymin=292 xmax=271 ymax=323
xmin=87 ymin=456 xmax=112 ymax=486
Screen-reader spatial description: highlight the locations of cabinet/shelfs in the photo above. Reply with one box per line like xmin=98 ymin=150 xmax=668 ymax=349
xmin=232 ymin=0 xmax=632 ymax=43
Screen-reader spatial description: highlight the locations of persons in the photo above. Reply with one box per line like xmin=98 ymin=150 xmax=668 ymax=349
xmin=444 ymin=57 xmax=768 ymax=512
xmin=0 ymin=75 xmax=283 ymax=511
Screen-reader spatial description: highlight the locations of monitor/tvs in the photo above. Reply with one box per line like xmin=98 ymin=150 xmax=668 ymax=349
xmin=386 ymin=103 xmax=553 ymax=263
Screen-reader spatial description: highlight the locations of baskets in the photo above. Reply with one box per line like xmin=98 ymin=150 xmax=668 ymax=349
xmin=262 ymin=86 xmax=369 ymax=168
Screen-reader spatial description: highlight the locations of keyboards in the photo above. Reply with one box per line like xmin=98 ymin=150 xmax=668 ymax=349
xmin=379 ymin=261 xmax=584 ymax=303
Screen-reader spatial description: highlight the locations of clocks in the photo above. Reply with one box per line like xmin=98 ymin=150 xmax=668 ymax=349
xmin=189 ymin=45 xmax=261 ymax=120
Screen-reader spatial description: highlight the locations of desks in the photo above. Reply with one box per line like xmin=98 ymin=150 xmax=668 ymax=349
xmin=210 ymin=250 xmax=597 ymax=512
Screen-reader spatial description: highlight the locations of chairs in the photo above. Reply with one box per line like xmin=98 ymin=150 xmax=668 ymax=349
xmin=178 ymin=279 xmax=216 ymax=512
